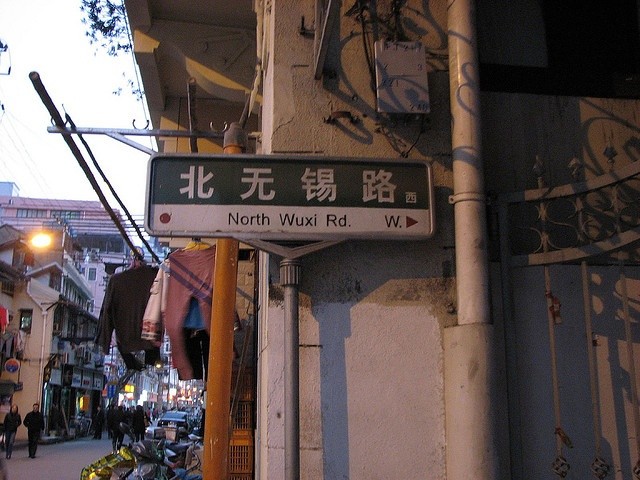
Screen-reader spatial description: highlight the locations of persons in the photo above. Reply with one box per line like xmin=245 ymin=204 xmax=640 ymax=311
xmin=107 ymin=403 xmax=145 ymax=451
xmin=185 ymin=407 xmax=190 ymax=413
xmin=2 ymin=403 xmax=21 ymax=457
xmin=152 ymin=408 xmax=156 ymax=419
xmin=172 ymin=407 xmax=177 ymax=412
xmin=181 ymin=406 xmax=185 ymax=412
xmin=23 ymin=404 xmax=44 ymax=459
xmin=91 ymin=404 xmax=104 ymax=440
xmin=147 ymin=408 xmax=150 ymax=420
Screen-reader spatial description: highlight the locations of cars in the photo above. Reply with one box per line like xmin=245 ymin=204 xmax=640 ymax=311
xmin=156 ymin=411 xmax=195 ymax=434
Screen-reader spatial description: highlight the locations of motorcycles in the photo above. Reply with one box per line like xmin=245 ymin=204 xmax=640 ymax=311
xmin=144 ymin=426 xmax=200 ymax=473
xmin=117 ymin=422 xmax=202 ymax=480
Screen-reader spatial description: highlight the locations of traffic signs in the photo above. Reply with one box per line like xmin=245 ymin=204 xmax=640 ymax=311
xmin=144 ymin=151 xmax=436 ymax=241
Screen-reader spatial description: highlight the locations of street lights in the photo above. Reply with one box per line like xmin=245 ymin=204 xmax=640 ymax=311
xmin=29 ymin=229 xmax=77 ymax=462
